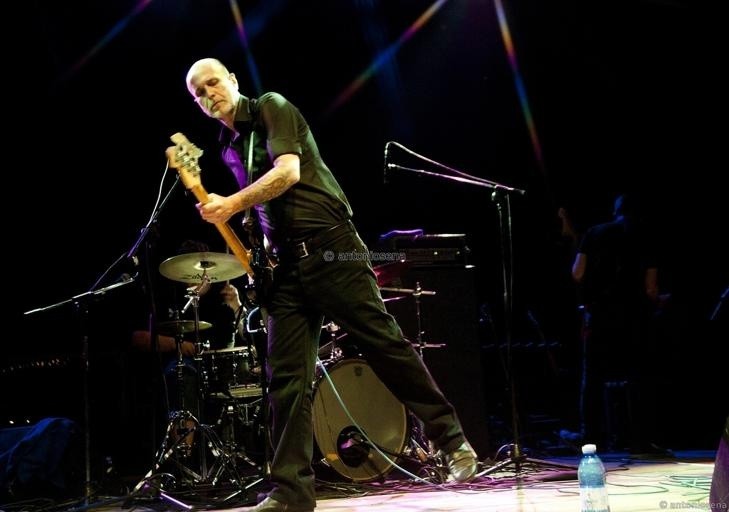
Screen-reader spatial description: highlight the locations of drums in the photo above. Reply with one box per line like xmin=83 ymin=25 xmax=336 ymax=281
xmin=202 ymin=346 xmax=259 ymax=401
xmin=309 ymin=358 xmax=413 ymax=486
xmin=317 ymin=325 xmax=355 ymax=361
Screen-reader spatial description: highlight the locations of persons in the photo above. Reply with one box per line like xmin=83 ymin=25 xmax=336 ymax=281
xmin=557 ymin=194 xmax=670 ymax=451
xmin=185 ymin=57 xmax=479 ymax=512
xmin=131 ymin=239 xmax=260 ymax=482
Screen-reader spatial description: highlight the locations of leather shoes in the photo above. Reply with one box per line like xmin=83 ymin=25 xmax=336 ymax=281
xmin=444 ymin=435 xmax=477 ymax=482
xmin=245 ymin=496 xmax=314 ymax=512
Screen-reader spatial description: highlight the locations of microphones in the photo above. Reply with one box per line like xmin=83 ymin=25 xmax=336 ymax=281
xmin=111 ymin=273 xmax=130 ymax=284
xmin=380 ymin=141 xmax=391 ymax=185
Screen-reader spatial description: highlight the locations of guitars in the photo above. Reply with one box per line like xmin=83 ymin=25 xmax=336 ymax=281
xmin=165 ymin=131 xmax=274 ymax=330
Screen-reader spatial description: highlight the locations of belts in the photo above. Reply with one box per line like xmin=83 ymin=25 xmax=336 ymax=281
xmin=292 ymin=219 xmax=355 ymax=258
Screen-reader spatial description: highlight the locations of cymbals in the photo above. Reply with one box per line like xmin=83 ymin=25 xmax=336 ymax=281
xmin=156 ymin=254 xmax=249 ymax=285
xmin=152 ymin=319 xmax=212 ymax=335
xmin=415 ymin=231 xmax=466 ymax=239
xmin=372 ymin=260 xmax=413 ymax=286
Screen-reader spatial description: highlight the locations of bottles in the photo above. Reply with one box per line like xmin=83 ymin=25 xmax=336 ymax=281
xmin=578 ymin=445 xmax=609 ymax=512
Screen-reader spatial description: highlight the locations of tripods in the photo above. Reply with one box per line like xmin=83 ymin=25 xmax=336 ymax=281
xmin=54 ymin=305 xmax=364 ymax=512
xmin=469 ymin=190 xmax=578 ymax=483
